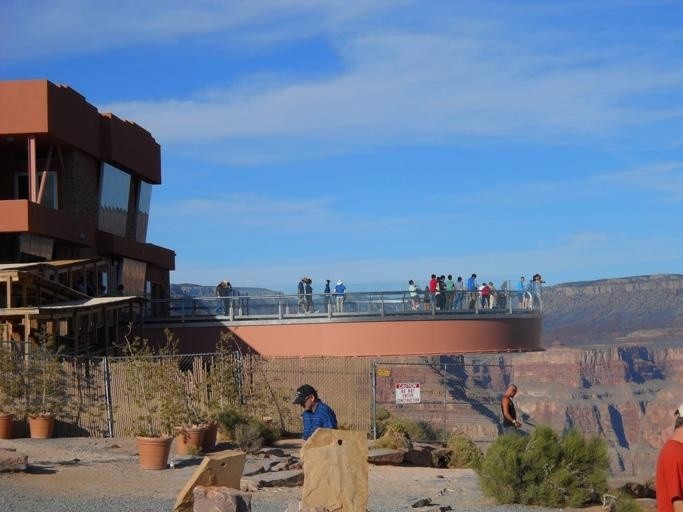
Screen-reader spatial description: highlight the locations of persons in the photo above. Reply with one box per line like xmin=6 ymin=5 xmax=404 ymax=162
xmin=303 ymin=279 xmax=319 ymax=314
xmin=653 ymin=404 xmax=683 ymax=512
xmin=333 ymin=279 xmax=347 ymax=314
xmin=428 ymin=273 xmax=546 ymax=312
xmin=322 ymin=279 xmax=331 ymax=315
xmin=221 ymin=282 xmax=232 ymax=314
xmin=213 ymin=281 xmax=225 ymax=314
xmin=296 ymin=277 xmax=309 ymax=314
xmin=407 ymin=278 xmax=421 ymax=313
xmin=291 ymin=383 xmax=337 ymax=446
xmin=499 ymin=383 xmax=524 ymax=437
xmin=422 ymin=286 xmax=430 ymax=312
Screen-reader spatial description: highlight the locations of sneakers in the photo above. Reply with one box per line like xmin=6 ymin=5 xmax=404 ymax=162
xmin=305 ymin=309 xmax=320 ymax=314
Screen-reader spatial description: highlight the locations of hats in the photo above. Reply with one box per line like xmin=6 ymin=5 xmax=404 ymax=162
xmin=292 ymin=384 xmax=315 ymax=404
xmin=302 ymin=278 xmax=309 ymax=282
xmin=337 ymin=280 xmax=343 ymax=285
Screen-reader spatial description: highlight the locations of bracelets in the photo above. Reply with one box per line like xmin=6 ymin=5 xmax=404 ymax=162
xmin=510 ymin=418 xmax=516 ymax=424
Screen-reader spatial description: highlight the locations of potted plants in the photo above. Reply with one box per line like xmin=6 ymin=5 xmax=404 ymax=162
xmin=110 ymin=320 xmax=217 ymax=469
xmin=0 ymin=326 xmax=65 ymax=439
